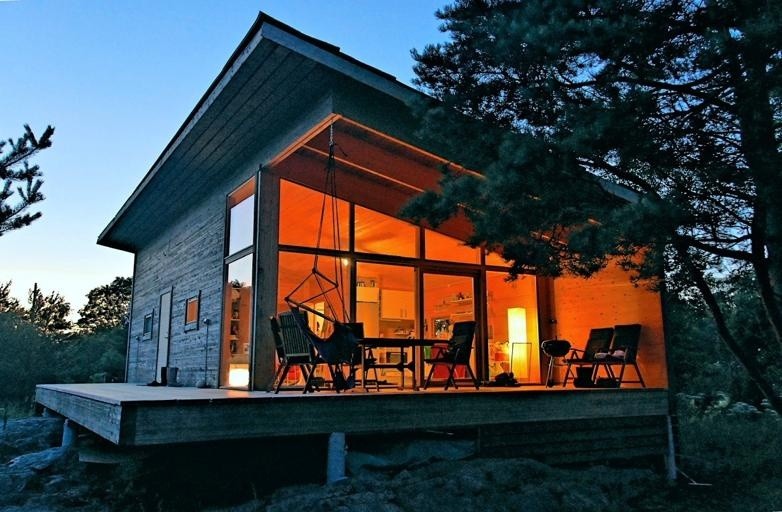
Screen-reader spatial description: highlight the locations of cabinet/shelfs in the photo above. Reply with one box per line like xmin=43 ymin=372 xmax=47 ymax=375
xmin=380 ymin=290 xmax=416 ymax=320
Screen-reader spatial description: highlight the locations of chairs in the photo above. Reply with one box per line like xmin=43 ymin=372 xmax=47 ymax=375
xmin=266 ymin=311 xmax=480 ymax=394
xmin=541 ymin=323 xmax=647 ymax=388
